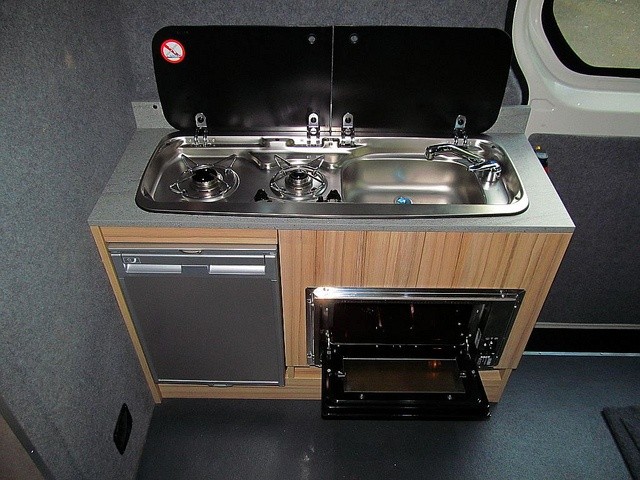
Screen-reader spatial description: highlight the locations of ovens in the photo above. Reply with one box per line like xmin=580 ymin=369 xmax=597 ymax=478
xmin=301 ymin=286 xmax=525 ymax=425
xmin=109 ymin=245 xmax=288 ymax=389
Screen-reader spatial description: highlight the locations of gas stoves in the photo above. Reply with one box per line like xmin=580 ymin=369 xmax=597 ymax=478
xmin=142 ymin=131 xmax=339 ymax=216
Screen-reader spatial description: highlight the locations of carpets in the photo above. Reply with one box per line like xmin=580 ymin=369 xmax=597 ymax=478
xmin=602 ymin=405 xmax=640 ymax=479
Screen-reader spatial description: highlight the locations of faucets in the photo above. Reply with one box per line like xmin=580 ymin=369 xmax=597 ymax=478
xmin=426 ymin=142 xmax=501 ymax=181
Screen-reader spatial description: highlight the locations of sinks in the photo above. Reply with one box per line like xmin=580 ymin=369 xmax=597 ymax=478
xmin=339 ymin=151 xmax=530 ymax=218
